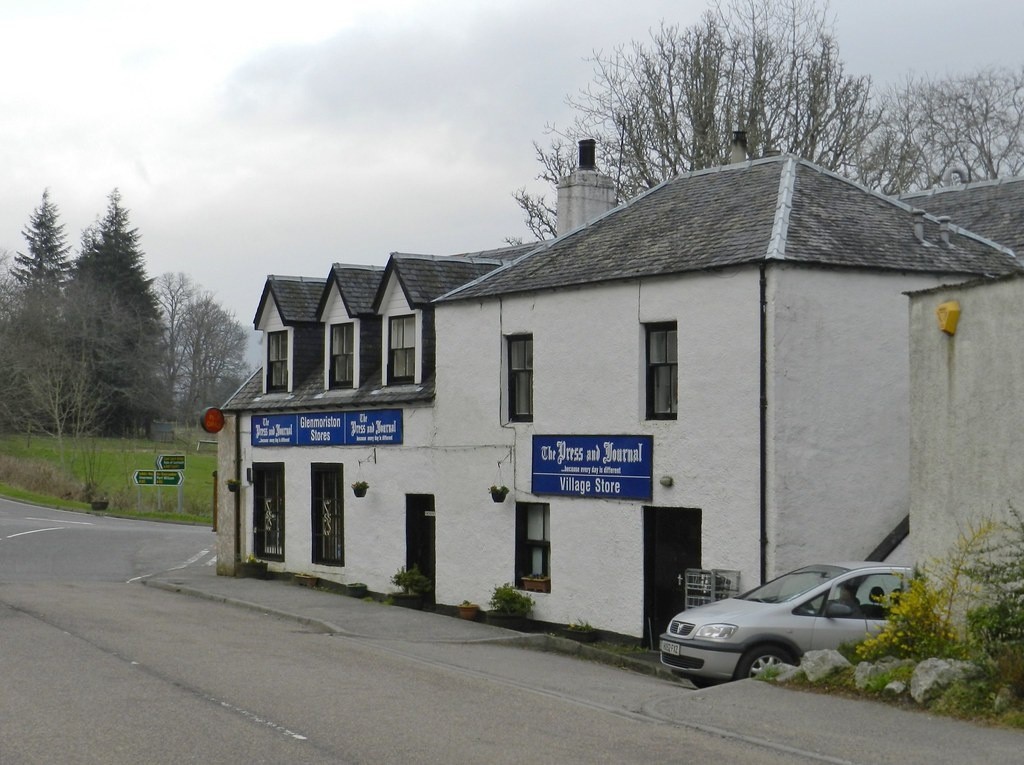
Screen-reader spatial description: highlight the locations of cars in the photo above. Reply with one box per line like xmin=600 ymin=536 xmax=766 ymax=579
xmin=659 ymin=563 xmax=913 ymax=688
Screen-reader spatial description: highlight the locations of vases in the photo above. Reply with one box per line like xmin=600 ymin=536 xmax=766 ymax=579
xmin=354 ymin=489 xmax=366 ymax=497
xmin=521 ymin=576 xmax=550 ymax=593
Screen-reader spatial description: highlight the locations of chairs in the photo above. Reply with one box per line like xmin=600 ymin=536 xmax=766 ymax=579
xmin=859 ymin=585 xmax=886 ymax=619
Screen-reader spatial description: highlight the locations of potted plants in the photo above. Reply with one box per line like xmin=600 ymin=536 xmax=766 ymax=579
xmin=347 ymin=583 xmax=367 ymax=599
xmin=564 ymin=628 xmax=599 ymax=642
xmin=294 ymin=571 xmax=320 ymax=588
xmin=485 ymin=581 xmax=535 ymax=629
xmin=488 ymin=485 xmax=510 ymax=503
xmin=458 ymin=599 xmax=478 ymax=621
xmin=234 ymin=552 xmax=268 ymax=579
xmin=391 ymin=563 xmax=433 ymax=609
xmin=224 ymin=479 xmax=241 ymax=492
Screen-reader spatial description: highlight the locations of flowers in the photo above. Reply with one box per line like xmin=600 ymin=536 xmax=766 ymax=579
xmin=351 ymin=481 xmax=369 ymax=491
xmin=530 ymin=573 xmax=544 ymax=580
xmin=567 ymin=616 xmax=593 ymax=631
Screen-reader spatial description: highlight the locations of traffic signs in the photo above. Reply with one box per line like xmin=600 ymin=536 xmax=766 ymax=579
xmin=155 ymin=470 xmax=185 ymax=486
xmin=155 ymin=455 xmax=186 ymax=470
xmin=132 ymin=470 xmax=154 ymax=486
